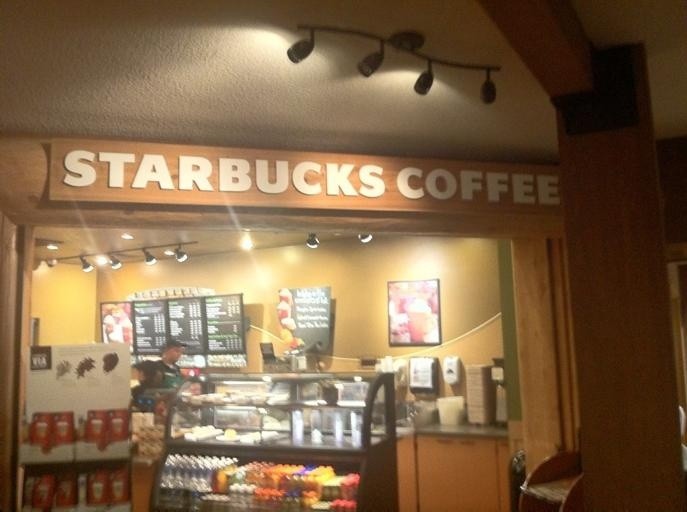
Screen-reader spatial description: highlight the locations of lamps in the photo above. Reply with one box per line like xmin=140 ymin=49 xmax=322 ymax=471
xmin=304 ymin=233 xmax=319 ymax=248
xmin=80 ymin=244 xmax=189 ymax=273
xmin=285 ymin=23 xmax=499 ymax=105
xmin=358 ymin=233 xmax=373 ymax=243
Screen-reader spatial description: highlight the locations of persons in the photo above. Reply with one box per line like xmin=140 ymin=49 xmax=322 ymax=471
xmin=131 ymin=340 xmax=185 ymax=412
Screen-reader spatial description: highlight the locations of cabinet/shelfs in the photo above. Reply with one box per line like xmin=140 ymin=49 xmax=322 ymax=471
xmin=148 ymin=368 xmax=399 ymax=511
xmin=517 ymin=449 xmax=584 ymax=510
xmin=395 ymin=432 xmax=511 ymax=512
xmin=15 ymin=341 xmax=132 ymax=511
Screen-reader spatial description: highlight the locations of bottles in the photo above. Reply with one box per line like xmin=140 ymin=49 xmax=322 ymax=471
xmin=162 ymin=455 xmax=359 ymax=512
xmin=311 ymin=409 xmax=324 ymax=448
xmin=331 ymin=409 xmax=345 ymax=448
xmin=291 ymin=408 xmax=306 ymax=448
xmin=349 ymin=409 xmax=363 ymax=448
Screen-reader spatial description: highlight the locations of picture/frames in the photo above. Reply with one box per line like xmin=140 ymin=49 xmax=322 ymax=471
xmin=387 ymin=278 xmax=442 ymax=346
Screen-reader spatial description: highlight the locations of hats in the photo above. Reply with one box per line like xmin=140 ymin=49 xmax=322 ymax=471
xmin=164 ymin=339 xmax=187 ymax=351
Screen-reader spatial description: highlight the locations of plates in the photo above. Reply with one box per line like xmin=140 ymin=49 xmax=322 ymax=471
xmin=216 ymin=435 xmax=241 ymax=443
